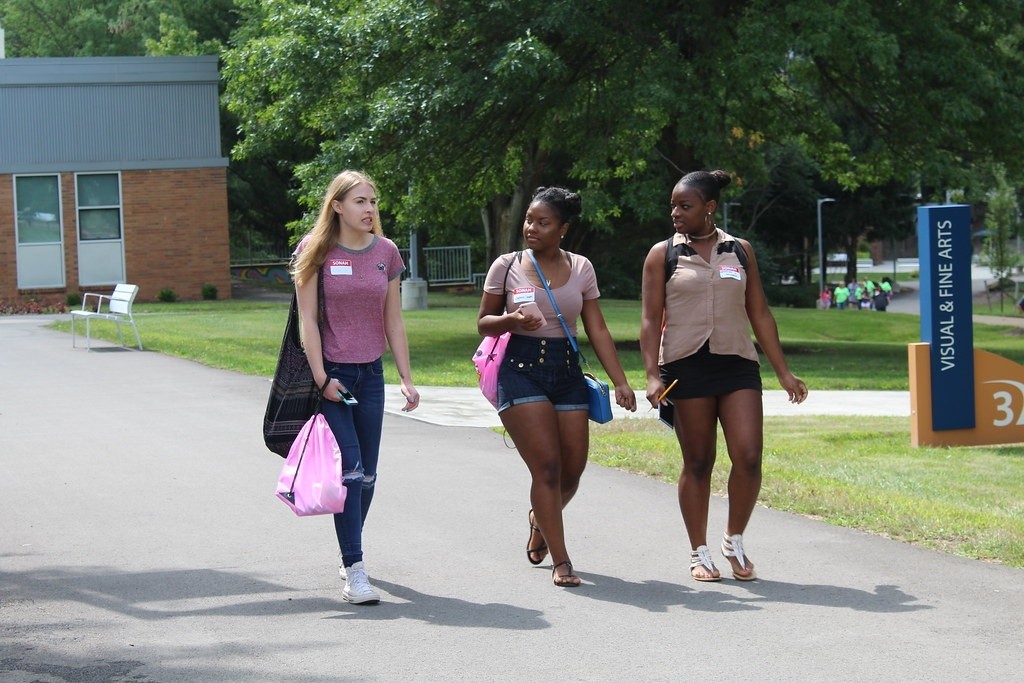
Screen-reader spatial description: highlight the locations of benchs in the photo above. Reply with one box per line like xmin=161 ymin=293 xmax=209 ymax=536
xmin=71 ymin=283 xmax=144 ymax=353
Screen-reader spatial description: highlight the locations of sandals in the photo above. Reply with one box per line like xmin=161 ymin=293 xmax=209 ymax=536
xmin=689 ymin=543 xmax=721 ymax=581
xmin=526 ymin=510 xmax=547 ymax=564
xmin=721 ymin=532 xmax=757 ymax=582
xmin=552 ymin=561 xmax=579 ymax=587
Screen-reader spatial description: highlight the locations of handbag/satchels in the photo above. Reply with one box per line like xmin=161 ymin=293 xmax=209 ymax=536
xmin=582 ymin=371 xmax=613 ymax=424
xmin=473 ymin=331 xmax=512 ymax=410
xmin=263 ymin=322 xmax=323 ymax=459
xmin=275 ymin=376 xmax=347 ymax=517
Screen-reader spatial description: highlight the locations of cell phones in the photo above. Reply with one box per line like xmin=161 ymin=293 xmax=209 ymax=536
xmin=657 ymin=397 xmax=676 ymax=430
xmin=334 ymin=379 xmax=358 ymax=406
xmin=518 ymin=302 xmax=547 ymax=327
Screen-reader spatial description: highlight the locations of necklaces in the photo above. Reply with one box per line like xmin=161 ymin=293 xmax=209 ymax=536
xmin=532 ymin=249 xmax=559 ymax=286
xmin=688 ymin=228 xmax=717 ymax=239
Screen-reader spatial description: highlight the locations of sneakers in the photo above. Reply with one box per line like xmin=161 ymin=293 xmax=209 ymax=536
xmin=337 ymin=548 xmax=346 ymax=580
xmin=342 ymin=561 xmax=380 ymax=604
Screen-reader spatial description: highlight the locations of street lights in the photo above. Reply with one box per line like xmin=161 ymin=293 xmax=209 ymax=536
xmin=817 ymin=198 xmax=837 ymax=292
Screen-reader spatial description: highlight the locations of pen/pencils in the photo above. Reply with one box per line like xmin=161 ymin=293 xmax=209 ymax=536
xmin=647 ymin=378 xmax=679 ymax=412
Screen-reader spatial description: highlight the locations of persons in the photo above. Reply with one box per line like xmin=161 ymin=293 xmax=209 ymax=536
xmin=292 ymin=171 xmax=420 ymax=604
xmin=639 ymin=170 xmax=809 ymax=581
xmin=477 ymin=187 xmax=637 ymax=587
xmin=819 ymin=276 xmax=893 ymax=312
